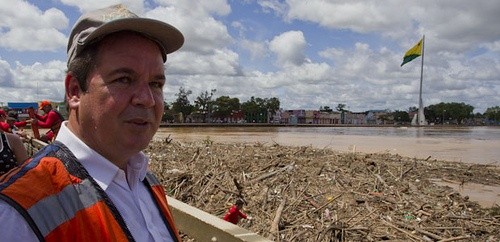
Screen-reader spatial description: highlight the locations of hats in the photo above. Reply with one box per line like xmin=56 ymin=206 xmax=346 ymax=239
xmin=39 ymin=101 xmax=51 ymax=110
xmin=66 ymin=5 xmax=185 ymax=67
xmin=0 ymin=110 xmax=7 ymax=116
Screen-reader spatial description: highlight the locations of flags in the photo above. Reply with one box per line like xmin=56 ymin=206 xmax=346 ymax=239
xmin=401 ymin=37 xmax=423 ymax=67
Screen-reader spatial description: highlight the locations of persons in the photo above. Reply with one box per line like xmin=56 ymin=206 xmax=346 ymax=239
xmin=0 ymin=101 xmax=64 ymax=177
xmin=223 ymin=200 xmax=253 ymax=224
xmin=0 ymin=4 xmax=185 ymax=242
